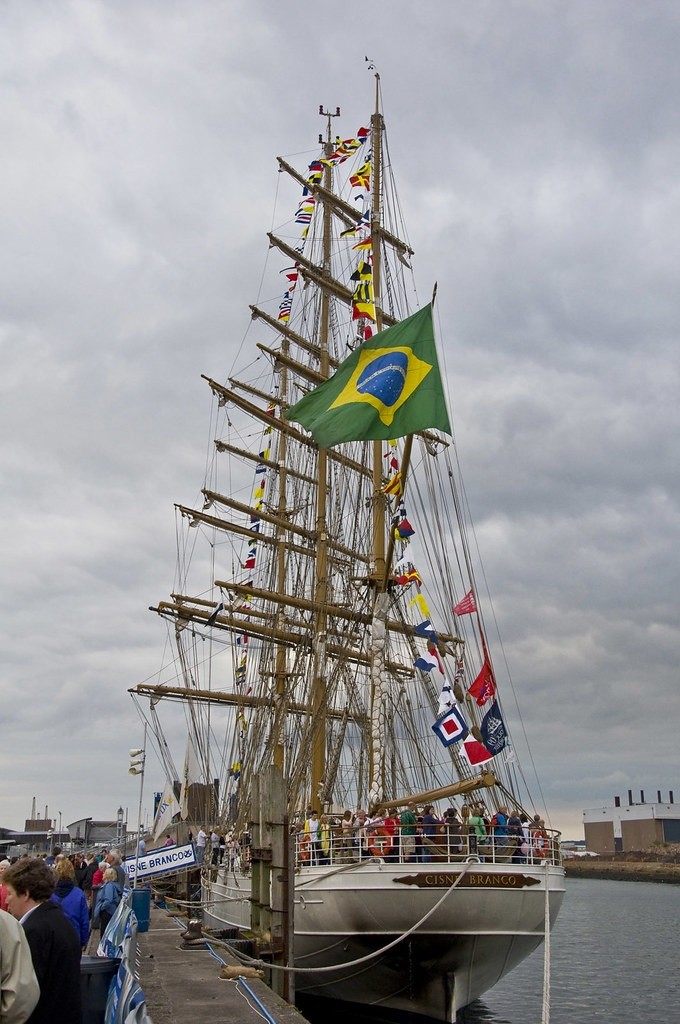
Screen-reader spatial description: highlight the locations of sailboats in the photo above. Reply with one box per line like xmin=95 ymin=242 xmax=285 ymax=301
xmin=127 ymin=69 xmax=566 ymax=1023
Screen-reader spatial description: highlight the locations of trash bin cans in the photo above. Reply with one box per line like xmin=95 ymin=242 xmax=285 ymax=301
xmin=133 ymin=889 xmax=150 ymax=933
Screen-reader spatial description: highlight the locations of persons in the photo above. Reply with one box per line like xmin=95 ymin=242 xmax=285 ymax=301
xmin=0 ymin=803 xmax=553 ymax=1024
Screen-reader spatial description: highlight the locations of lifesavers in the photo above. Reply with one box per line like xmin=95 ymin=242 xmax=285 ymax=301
xmin=369 ymin=828 xmax=391 ymax=855
xmin=300 ymin=835 xmax=311 ymax=859
xmin=533 ymin=831 xmax=548 ymax=857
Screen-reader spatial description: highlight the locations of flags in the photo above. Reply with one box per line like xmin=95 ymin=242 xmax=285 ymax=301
xmin=376 ymin=439 xmax=509 ymax=768
xmin=150 ymin=778 xmax=180 ymax=841
xmin=277 ymin=125 xmax=375 ymax=337
xmin=179 ymin=733 xmax=202 ymax=821
xmin=227 ymin=393 xmax=278 ymax=781
xmin=283 ymin=303 xmax=452 ymax=448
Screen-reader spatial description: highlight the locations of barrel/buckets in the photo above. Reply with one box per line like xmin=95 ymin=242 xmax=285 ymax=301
xmin=132 ymin=888 xmax=151 ymax=932
xmin=80 ymin=954 xmax=114 ymax=1024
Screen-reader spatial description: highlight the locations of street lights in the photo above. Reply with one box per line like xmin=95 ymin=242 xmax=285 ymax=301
xmin=128 ymin=746 xmax=146 ymax=889
xmin=47 ymin=829 xmax=54 ymax=853
